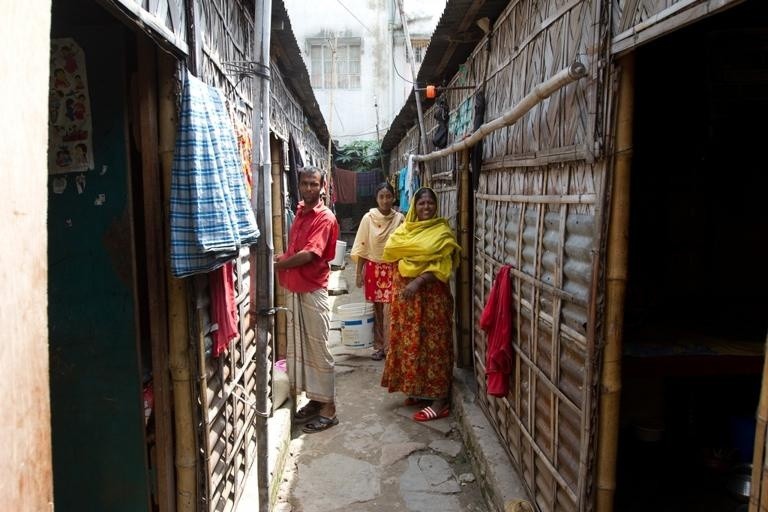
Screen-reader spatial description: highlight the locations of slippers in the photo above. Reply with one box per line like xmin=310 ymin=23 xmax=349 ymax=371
xmin=405 ymin=398 xmax=421 ymax=406
xmin=415 ymin=406 xmax=449 ymax=420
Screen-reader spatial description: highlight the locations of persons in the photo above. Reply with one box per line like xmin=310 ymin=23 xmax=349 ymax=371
xmin=377 ymin=186 xmax=464 ymax=422
xmin=272 ymin=166 xmax=340 ymax=435
xmin=347 ymin=183 xmax=407 ymax=361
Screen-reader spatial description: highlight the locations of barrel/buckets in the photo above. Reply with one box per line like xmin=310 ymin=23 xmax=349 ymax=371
xmin=339 ymin=281 xmax=375 ymax=350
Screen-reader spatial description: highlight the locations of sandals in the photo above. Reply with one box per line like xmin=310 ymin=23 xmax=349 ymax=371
xmin=295 ymin=400 xmax=339 ymax=433
xmin=372 ymin=349 xmax=384 ymax=360
